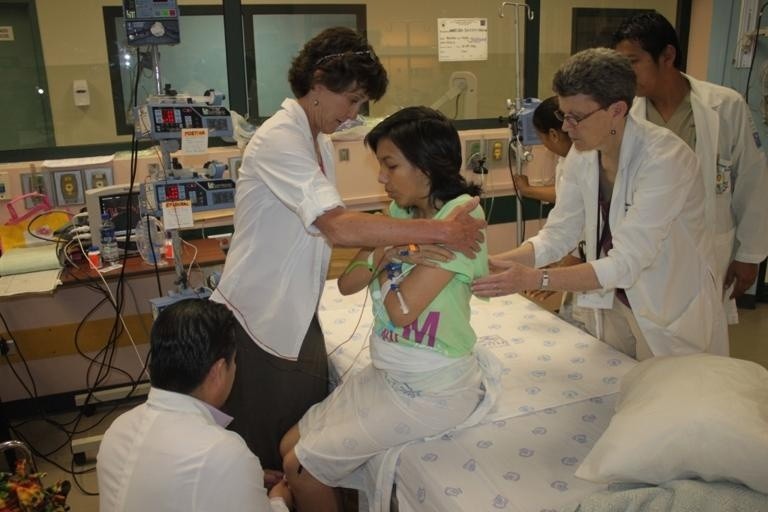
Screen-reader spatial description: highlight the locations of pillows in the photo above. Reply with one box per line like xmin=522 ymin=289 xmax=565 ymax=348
xmin=571 ymin=351 xmax=768 ymax=495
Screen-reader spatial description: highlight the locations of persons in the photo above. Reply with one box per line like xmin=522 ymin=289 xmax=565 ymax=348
xmin=278 ymin=106 xmax=490 ymax=512
xmin=207 ymin=29 xmax=489 ymax=512
xmin=469 ymin=48 xmax=729 ymax=363
xmin=518 ymin=11 xmax=767 ymax=327
xmin=96 ymin=297 xmax=294 ymax=512
xmin=512 ymin=96 xmax=572 ymax=205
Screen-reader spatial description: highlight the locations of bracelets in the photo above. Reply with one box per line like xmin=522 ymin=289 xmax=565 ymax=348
xmin=538 ymin=268 xmax=548 ymax=290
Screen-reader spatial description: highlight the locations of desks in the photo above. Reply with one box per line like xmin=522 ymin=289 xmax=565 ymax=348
xmin=56 ymin=229 xmax=236 ymax=290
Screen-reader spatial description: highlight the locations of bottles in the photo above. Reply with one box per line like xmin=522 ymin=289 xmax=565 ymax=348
xmin=87 ymin=246 xmax=102 ymax=269
xmin=99 ymin=211 xmax=120 ymax=266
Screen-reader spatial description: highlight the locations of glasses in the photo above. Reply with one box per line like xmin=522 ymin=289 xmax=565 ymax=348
xmin=554 ymin=107 xmax=608 ymax=126
xmin=316 ymin=50 xmax=376 ymax=66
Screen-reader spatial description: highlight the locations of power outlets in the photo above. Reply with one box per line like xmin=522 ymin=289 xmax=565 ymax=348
xmin=1 ymin=339 xmax=16 ymax=357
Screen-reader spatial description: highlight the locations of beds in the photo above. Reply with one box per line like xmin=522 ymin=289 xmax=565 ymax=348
xmin=314 ymin=269 xmax=646 ymax=511
